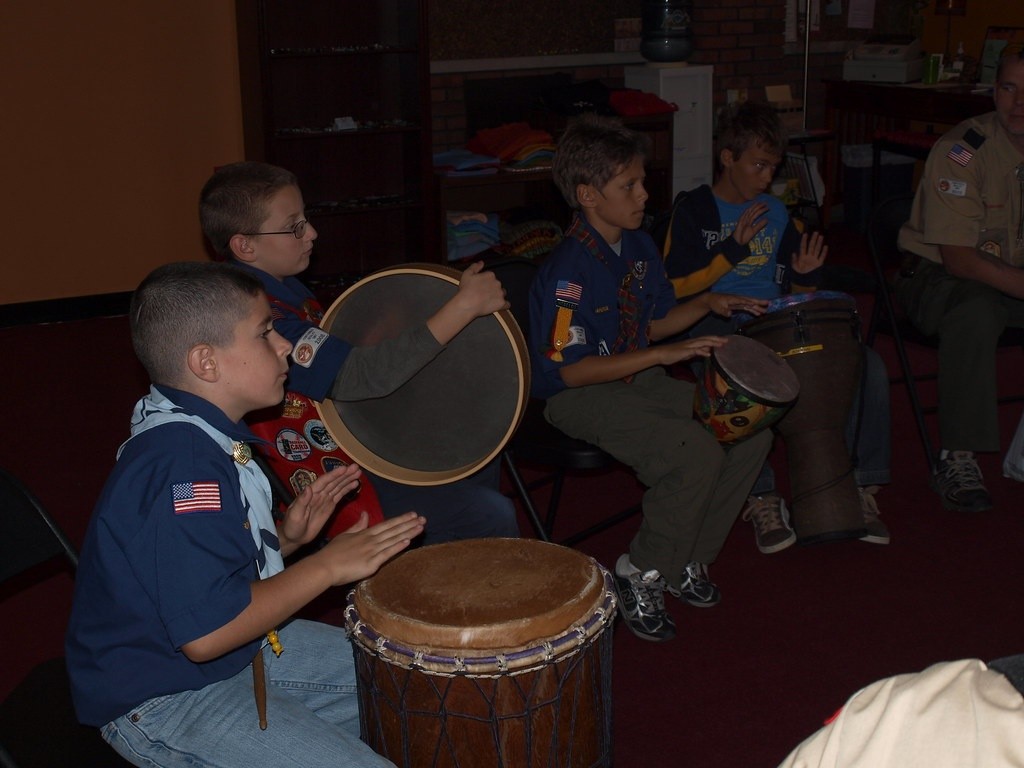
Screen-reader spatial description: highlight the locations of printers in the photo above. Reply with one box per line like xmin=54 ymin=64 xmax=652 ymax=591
xmin=843 ymin=33 xmax=924 ymax=83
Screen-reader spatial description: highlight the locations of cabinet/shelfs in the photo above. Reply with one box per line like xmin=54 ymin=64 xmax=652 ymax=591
xmin=260 ymin=0 xmax=430 ymax=303
xmin=429 ymin=50 xmax=651 ymax=272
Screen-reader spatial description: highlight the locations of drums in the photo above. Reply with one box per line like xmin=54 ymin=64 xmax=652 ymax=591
xmin=733 ymin=289 xmax=866 ymax=541
xmin=691 ymin=335 xmax=799 ymax=443
xmin=314 ymin=263 xmax=530 ymax=486
xmin=347 ymin=537 xmax=619 ymax=768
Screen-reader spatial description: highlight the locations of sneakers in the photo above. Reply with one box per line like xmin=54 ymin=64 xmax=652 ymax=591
xmin=932 ymin=445 xmax=993 ymax=514
xmin=743 ymin=492 xmax=795 ymax=554
xmin=667 ymin=560 xmax=720 ymax=608
xmin=609 ymin=566 xmax=677 ymax=641
xmin=856 ymin=486 xmax=890 ymax=545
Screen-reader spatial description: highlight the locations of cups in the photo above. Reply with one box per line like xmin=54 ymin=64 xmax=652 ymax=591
xmin=921 ymin=55 xmax=941 ymax=84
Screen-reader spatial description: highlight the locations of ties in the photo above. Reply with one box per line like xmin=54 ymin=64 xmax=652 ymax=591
xmin=117 ymin=387 xmax=284 ymax=648
xmin=569 ymin=218 xmax=653 ymax=384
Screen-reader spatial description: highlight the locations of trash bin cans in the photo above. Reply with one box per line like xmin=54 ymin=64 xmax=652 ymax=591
xmin=842 ymin=144 xmax=916 ymax=237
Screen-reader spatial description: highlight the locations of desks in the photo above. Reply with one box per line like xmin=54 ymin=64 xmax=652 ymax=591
xmin=829 ymin=75 xmax=995 ymax=130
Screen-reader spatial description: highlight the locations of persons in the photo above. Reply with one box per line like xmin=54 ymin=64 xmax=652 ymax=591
xmin=199 ymin=161 xmax=520 ymax=553
xmin=66 ymin=261 xmax=447 ymax=768
xmin=895 ymin=39 xmax=1024 ymax=513
xmin=661 ymin=105 xmax=892 ymax=554
xmin=524 ymin=114 xmax=771 ymax=642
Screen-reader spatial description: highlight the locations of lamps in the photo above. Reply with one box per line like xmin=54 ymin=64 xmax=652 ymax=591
xmin=935 ymin=1 xmax=966 ymax=49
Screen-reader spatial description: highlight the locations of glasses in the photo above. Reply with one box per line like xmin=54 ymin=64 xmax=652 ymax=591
xmin=226 ymin=218 xmax=308 ymax=244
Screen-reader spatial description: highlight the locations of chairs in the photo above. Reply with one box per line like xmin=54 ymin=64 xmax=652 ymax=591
xmin=468 ymin=258 xmax=646 ymax=550
xmin=837 ymin=162 xmax=1024 ymax=476
xmin=1 ymin=471 xmax=136 ymax=768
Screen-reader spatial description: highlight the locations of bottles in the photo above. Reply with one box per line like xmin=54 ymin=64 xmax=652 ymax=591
xmin=640 ymin=0 xmax=694 ymax=61
xmin=739 ymin=76 xmax=752 ymax=103
